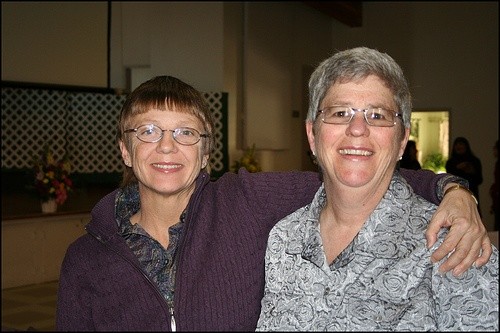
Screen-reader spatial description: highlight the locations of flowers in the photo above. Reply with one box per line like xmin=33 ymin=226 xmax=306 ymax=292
xmin=231 ymin=142 xmax=264 ymax=174
xmin=33 ymin=131 xmax=78 ymax=205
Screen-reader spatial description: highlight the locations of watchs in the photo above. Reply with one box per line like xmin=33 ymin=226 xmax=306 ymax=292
xmin=443 ymin=184 xmax=478 ymax=206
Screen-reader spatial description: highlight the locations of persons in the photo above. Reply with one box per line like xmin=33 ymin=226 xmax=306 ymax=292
xmin=445 ymin=137 xmax=483 ymax=220
xmin=489 ymin=139 xmax=500 ymax=232
xmin=255 ymin=47 xmax=500 ymax=333
xmin=56 ymin=75 xmax=492 ymax=333
xmin=397 ymin=140 xmax=421 ymax=171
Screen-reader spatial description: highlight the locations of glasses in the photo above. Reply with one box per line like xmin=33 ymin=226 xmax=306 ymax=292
xmin=124 ymin=123 xmax=207 ymax=146
xmin=318 ymin=106 xmax=402 ymax=127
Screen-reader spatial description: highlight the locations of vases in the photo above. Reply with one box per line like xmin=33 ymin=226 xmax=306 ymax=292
xmin=40 ymin=188 xmax=58 ymax=213
